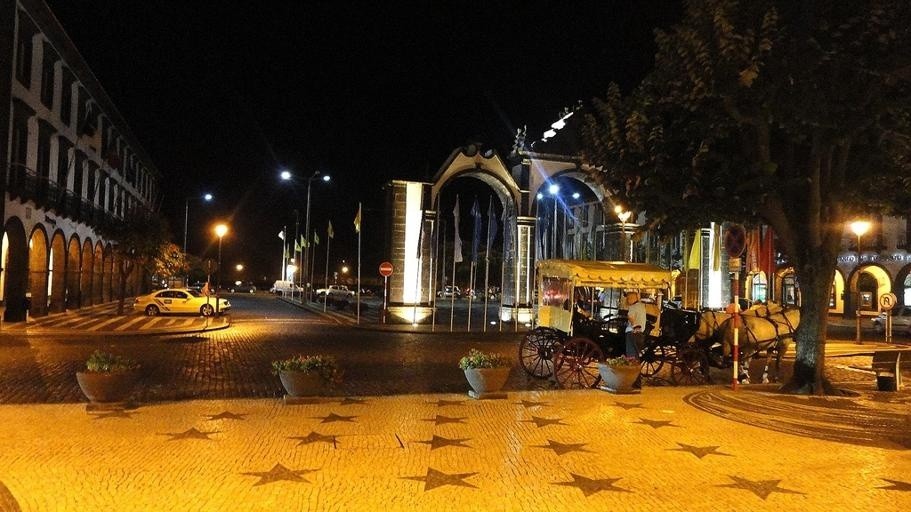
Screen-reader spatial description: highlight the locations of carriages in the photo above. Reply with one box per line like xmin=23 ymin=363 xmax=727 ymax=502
xmin=517 ymin=257 xmax=800 ymax=387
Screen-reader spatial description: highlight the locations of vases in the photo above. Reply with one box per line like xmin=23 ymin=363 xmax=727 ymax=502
xmin=464 ymin=368 xmax=511 ymax=392
xmin=278 ymin=371 xmax=325 ymax=395
xmin=599 ymin=365 xmax=640 ymax=388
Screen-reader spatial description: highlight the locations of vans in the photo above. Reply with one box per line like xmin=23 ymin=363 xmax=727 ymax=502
xmin=437 ymin=285 xmax=462 ymax=298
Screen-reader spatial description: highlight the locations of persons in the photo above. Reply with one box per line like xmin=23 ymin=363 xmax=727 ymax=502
xmin=562 ymin=289 xmax=613 ymax=358
xmin=623 ymin=292 xmax=647 ymax=390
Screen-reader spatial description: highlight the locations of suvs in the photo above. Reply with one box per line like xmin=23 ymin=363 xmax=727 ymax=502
xmin=270 ymin=279 xmax=304 ymax=295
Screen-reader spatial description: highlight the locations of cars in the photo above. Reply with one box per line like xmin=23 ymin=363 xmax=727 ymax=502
xmin=316 ymin=289 xmax=329 ymax=296
xmin=133 ymin=288 xmax=232 ymax=317
xmin=192 ymin=283 xmax=215 ymax=295
xmin=326 ymin=284 xmax=356 ymax=297
xmin=151 ymin=279 xmax=167 ymax=290
xmin=227 ymin=283 xmax=257 ymax=294
xmin=870 ymin=305 xmax=910 ymax=337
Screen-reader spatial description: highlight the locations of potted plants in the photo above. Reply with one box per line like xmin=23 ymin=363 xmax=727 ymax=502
xmin=77 ymin=350 xmax=142 ymax=402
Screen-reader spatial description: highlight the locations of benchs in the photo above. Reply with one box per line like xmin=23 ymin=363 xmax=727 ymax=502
xmin=872 ymin=350 xmax=911 ymax=391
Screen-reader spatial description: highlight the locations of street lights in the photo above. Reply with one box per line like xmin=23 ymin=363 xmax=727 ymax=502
xmin=281 ymin=170 xmax=332 ymax=304
xmin=846 ymin=216 xmax=873 ymax=344
xmin=183 ymin=193 xmax=213 ymax=258
xmin=538 ymin=182 xmax=582 ymax=259
xmin=213 ymin=224 xmax=230 ymax=318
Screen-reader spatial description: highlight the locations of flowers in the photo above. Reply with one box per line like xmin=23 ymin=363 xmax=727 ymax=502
xmin=596 ymin=356 xmax=643 ymax=369
xmin=457 ymin=349 xmax=515 ymax=372
xmin=273 ymin=356 xmax=344 ymax=384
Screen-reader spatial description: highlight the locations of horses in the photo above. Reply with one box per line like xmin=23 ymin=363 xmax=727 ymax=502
xmin=693 ymin=300 xmax=801 ymax=384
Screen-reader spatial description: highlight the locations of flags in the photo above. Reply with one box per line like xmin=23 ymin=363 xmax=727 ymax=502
xmin=352 ymin=205 xmax=362 ymax=234
xmin=281 ymin=231 xmax=320 ymax=259
xmin=327 ymin=221 xmax=335 ymax=239
xmin=415 ymin=189 xmax=513 ymax=265
